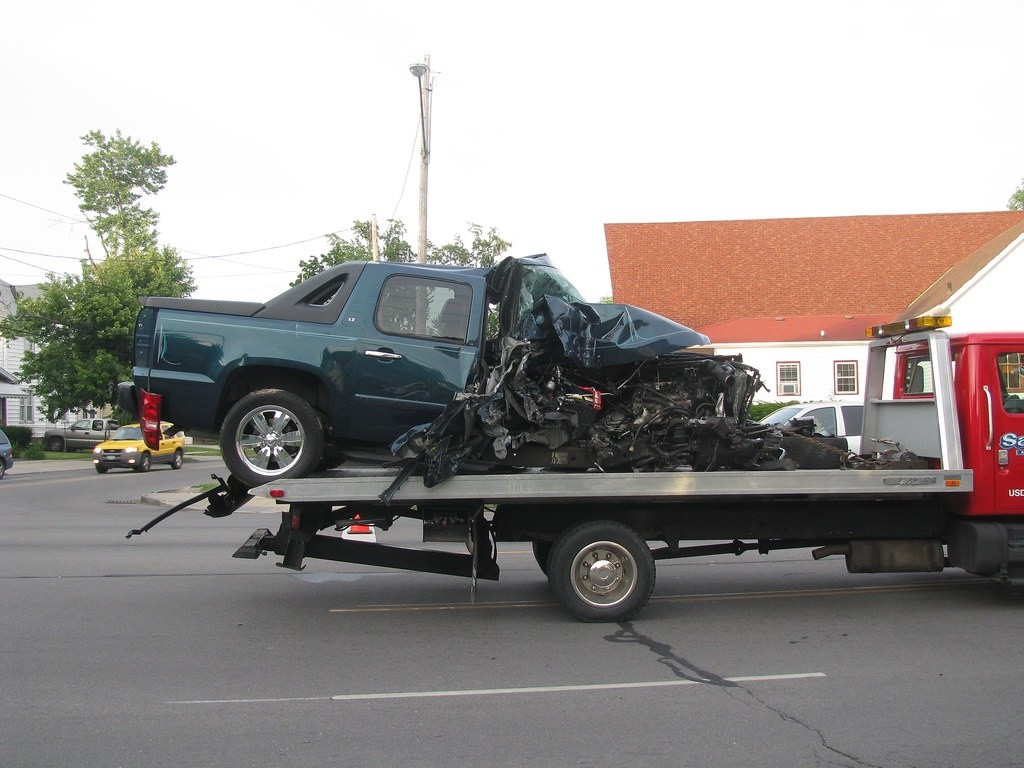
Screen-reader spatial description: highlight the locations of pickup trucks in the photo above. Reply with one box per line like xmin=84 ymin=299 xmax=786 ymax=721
xmin=116 ymin=260 xmax=755 ymax=488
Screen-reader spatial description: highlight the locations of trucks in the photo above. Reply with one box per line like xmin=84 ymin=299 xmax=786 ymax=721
xmin=231 ymin=316 xmax=1024 ymax=626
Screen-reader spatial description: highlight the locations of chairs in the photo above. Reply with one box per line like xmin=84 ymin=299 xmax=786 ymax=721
xmin=908 ymin=364 xmax=927 ymax=393
xmin=438 ymin=293 xmax=470 ymax=340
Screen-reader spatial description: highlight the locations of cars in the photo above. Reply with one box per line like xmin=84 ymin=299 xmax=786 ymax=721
xmin=758 ymin=398 xmax=864 ymax=458
xmin=0 ymin=429 xmax=14 ymax=480
xmin=44 ymin=418 xmax=122 ymax=452
xmin=93 ymin=421 xmax=187 ymax=474
